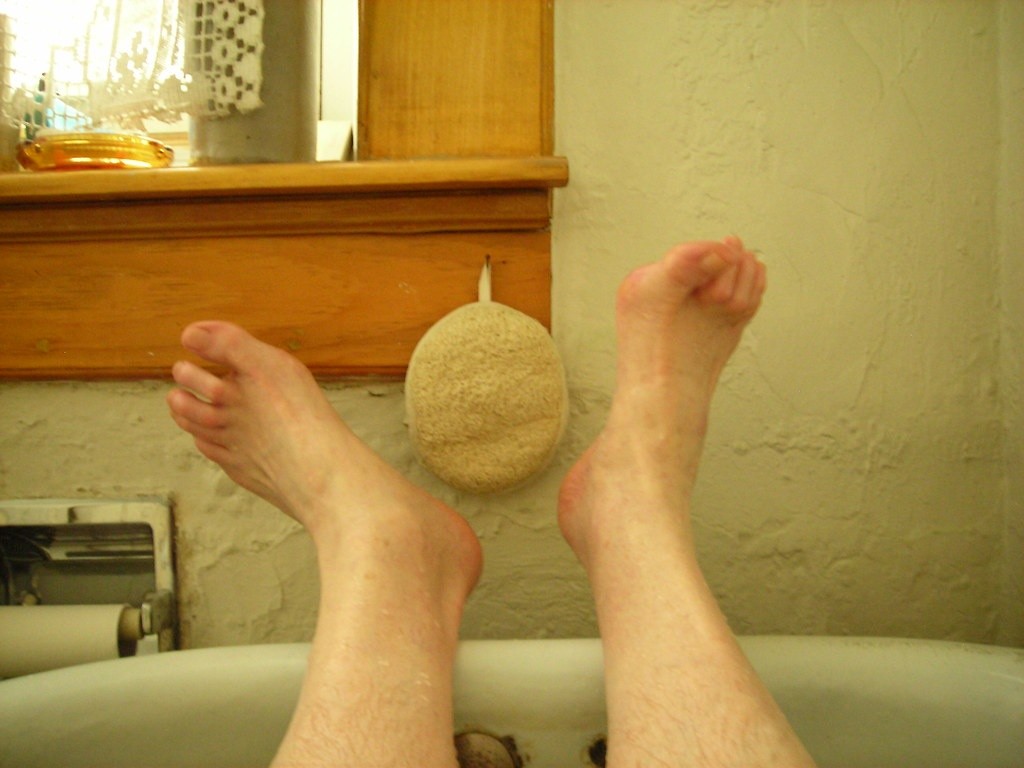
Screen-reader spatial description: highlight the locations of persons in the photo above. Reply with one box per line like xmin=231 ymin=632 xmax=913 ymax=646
xmin=166 ymin=238 xmax=819 ymax=768
